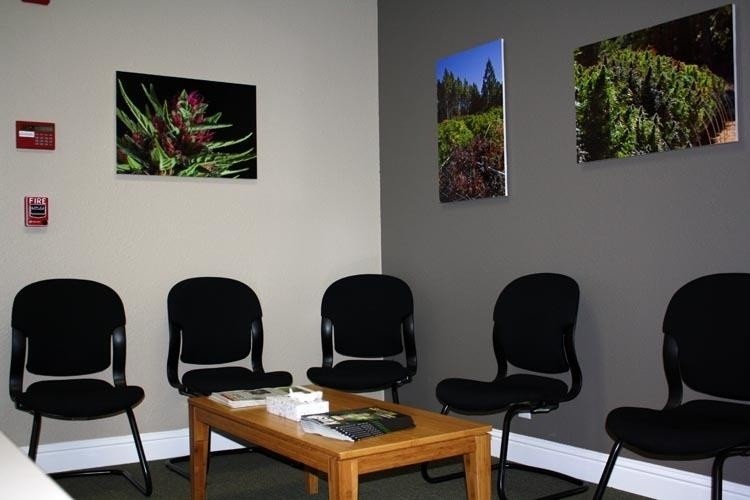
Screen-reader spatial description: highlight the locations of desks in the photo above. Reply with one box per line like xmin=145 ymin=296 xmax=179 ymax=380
xmin=188 ymin=384 xmax=493 ymax=500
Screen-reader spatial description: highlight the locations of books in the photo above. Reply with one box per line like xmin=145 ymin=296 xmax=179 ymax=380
xmin=300 ymin=405 xmax=417 ymax=442
xmin=208 ymin=386 xmax=316 ymax=409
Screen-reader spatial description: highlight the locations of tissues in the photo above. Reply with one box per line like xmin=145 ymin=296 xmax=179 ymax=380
xmin=263 ymin=387 xmax=331 ymax=421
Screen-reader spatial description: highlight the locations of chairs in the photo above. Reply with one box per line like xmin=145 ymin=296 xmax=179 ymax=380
xmin=594 ymin=273 xmax=749 ymax=500
xmin=307 ymin=274 xmax=418 ymax=404
xmin=165 ymin=275 xmax=293 ymax=481
xmin=9 ymin=278 xmax=153 ymax=496
xmin=422 ymin=273 xmax=588 ymax=499
